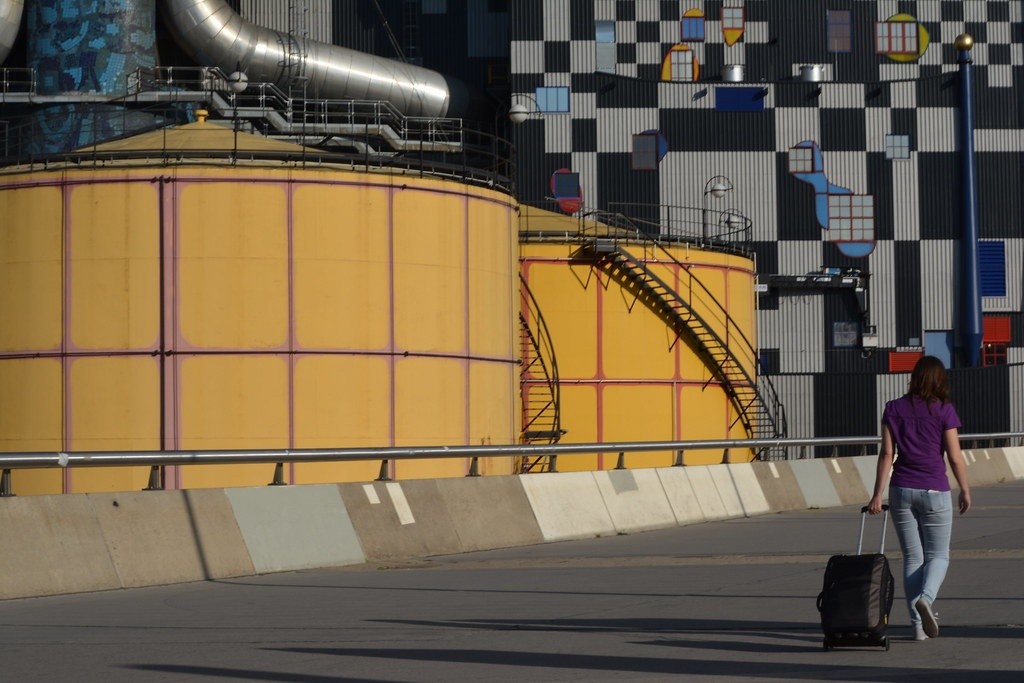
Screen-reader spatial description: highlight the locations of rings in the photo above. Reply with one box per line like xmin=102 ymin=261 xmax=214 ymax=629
xmin=873 ymin=512 xmax=875 ymax=514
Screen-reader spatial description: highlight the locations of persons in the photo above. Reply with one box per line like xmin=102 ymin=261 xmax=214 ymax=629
xmin=867 ymin=355 xmax=970 ymax=641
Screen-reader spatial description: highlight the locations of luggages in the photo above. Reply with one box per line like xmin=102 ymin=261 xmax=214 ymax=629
xmin=816 ymin=504 xmax=895 ymax=652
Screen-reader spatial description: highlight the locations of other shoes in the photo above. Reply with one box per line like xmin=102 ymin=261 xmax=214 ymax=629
xmin=914 ymin=597 xmax=939 ymax=641
